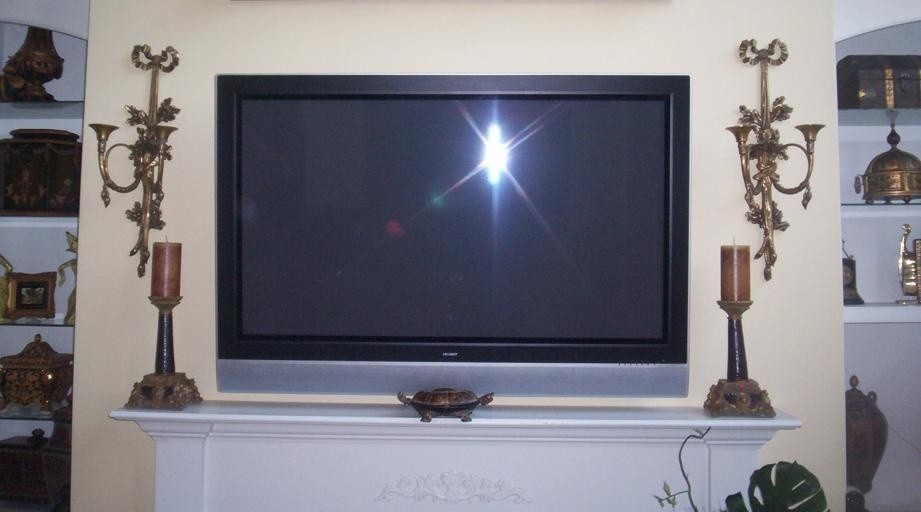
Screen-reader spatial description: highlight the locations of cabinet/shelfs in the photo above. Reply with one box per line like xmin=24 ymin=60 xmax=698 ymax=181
xmin=0 ymin=21 xmax=90 ymax=512
xmin=835 ymin=19 xmax=921 ymax=511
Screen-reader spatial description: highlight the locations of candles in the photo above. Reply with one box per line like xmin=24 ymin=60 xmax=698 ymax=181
xmin=720 ymin=239 xmax=751 ymax=302
xmin=151 ymin=235 xmax=182 ymax=298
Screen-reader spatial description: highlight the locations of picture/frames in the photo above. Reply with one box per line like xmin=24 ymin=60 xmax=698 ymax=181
xmin=4 ymin=271 xmax=57 ymax=320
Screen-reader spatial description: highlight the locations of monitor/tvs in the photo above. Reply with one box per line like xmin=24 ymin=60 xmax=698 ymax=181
xmin=215 ymin=72 xmax=692 ymax=399
